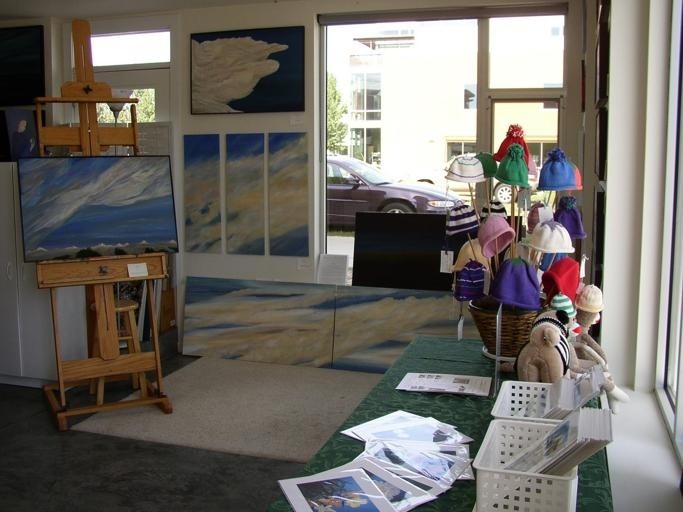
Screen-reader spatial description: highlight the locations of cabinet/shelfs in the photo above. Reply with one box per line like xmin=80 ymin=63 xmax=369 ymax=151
xmin=0 ymin=162 xmax=90 ymax=392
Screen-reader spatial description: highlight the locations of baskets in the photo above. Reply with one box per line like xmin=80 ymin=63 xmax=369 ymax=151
xmin=490 ymin=379 xmax=583 ymax=423
xmin=472 ymin=418 xmax=581 ymax=511
xmin=467 ymin=292 xmax=550 ymax=359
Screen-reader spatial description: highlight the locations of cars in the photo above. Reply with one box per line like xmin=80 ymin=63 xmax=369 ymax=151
xmin=325 ymin=151 xmax=463 ymax=229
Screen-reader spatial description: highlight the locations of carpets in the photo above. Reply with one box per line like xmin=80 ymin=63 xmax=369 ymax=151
xmin=68 ymin=355 xmax=385 ymax=463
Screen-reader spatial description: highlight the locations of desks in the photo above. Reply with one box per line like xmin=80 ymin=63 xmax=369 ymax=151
xmin=266 ymin=335 xmax=615 ymax=512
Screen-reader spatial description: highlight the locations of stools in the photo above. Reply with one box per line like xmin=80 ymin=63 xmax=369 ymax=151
xmin=89 ymin=301 xmax=148 ymax=405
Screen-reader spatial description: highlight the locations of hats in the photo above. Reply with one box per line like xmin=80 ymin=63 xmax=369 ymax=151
xmin=454 ymin=258 xmax=494 ymax=303
xmin=445 ymin=199 xmax=481 ymax=235
xmin=472 ymin=151 xmax=499 ymax=178
xmin=491 ymin=123 xmax=531 ymax=171
xmin=541 ymin=255 xmax=581 ymax=307
xmin=567 ymin=164 xmax=583 ymax=191
xmin=552 ymin=195 xmax=588 ymax=241
xmin=526 ymin=200 xmax=554 ymax=235
xmin=480 ymin=198 xmax=508 ymax=224
xmin=451 ymin=237 xmax=494 ymax=272
xmin=527 ymin=219 xmax=576 ymax=256
xmin=445 ymin=153 xmax=487 ymax=183
xmin=475 ymin=213 xmax=515 ymax=257
xmin=536 ymin=147 xmax=577 ymax=192
xmin=490 ymin=254 xmax=542 ymax=310
xmin=494 ymin=145 xmax=531 ymax=188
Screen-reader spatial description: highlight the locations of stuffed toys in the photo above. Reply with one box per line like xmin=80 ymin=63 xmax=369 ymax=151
xmin=517 ymin=283 xmax=630 ymax=415
